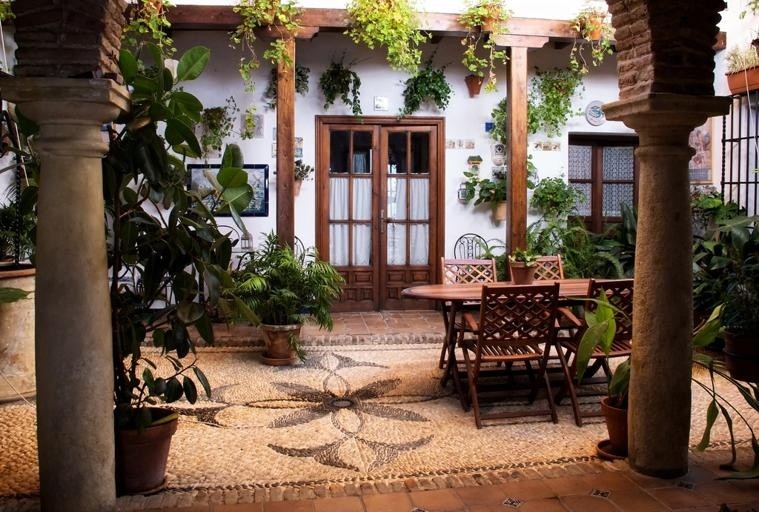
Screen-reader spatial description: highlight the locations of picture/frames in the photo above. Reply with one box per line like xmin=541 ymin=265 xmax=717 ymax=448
xmin=687 ymin=117 xmax=715 ymax=185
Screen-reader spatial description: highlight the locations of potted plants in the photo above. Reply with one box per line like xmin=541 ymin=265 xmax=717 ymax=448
xmin=693 ymin=223 xmax=759 ymax=383
xmin=457 ymin=0 xmax=613 ymax=94
xmin=463 ymin=154 xmax=536 ymax=221
xmin=725 ymin=46 xmax=759 ymax=95
xmin=565 ymin=290 xmax=759 ymax=482
